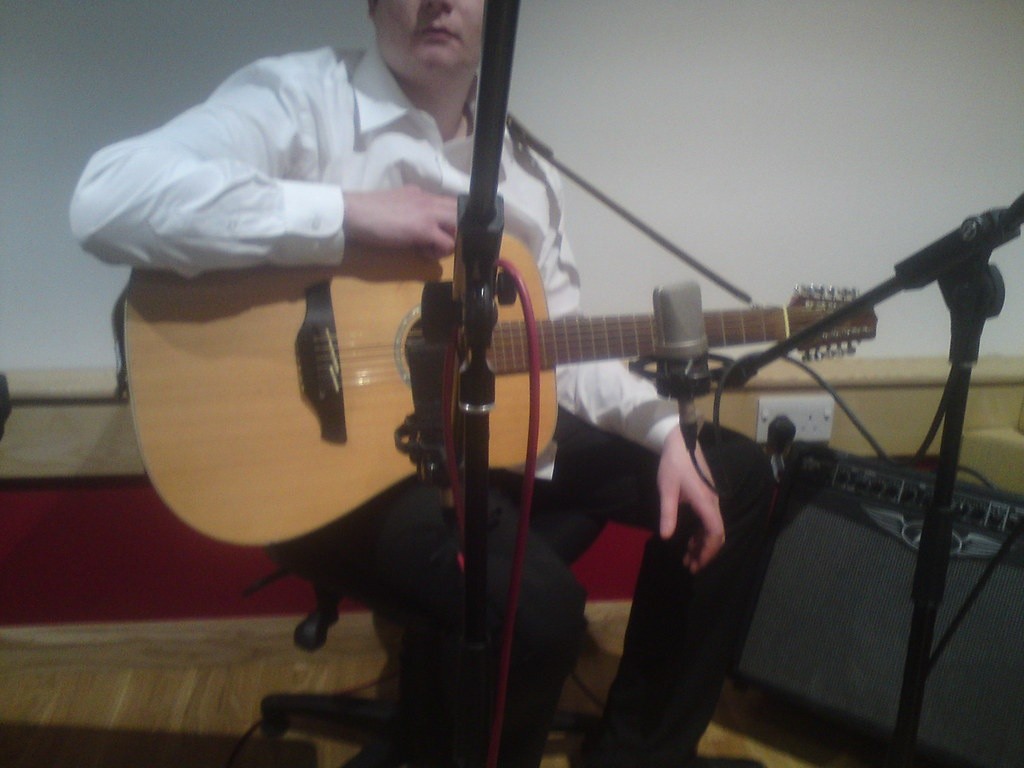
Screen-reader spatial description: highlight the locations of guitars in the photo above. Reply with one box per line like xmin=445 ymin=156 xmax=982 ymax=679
xmin=117 ymin=223 xmax=876 ymax=548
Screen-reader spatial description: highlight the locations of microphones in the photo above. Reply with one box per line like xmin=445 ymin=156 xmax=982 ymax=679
xmin=651 ymin=280 xmax=712 ymax=398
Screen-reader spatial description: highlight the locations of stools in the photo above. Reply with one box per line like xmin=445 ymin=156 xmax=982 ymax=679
xmin=260 ymin=507 xmax=607 ymax=768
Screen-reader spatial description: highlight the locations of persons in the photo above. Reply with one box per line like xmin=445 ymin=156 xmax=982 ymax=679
xmin=67 ymin=0 xmax=773 ymax=768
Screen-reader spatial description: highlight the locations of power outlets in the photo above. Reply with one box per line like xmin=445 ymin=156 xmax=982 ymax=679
xmin=758 ymin=397 xmax=836 ymax=443
